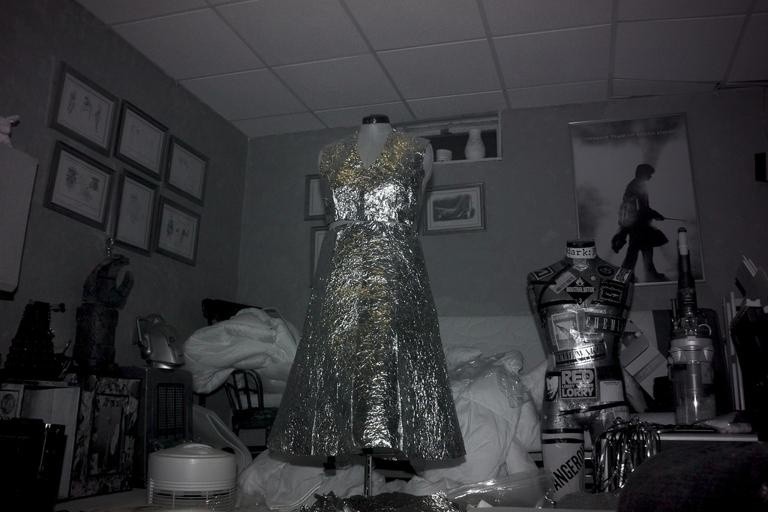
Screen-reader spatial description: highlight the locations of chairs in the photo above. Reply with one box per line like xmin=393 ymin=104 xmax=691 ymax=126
xmin=556 ymin=443 xmax=767 ymax=512
xmin=200 ymin=297 xmax=283 ymax=455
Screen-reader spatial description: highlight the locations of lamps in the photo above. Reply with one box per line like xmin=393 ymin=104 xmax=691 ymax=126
xmin=134 ymin=310 xmax=173 ymax=370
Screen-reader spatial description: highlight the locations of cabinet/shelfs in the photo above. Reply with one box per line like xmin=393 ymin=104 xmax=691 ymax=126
xmin=112 ymin=361 xmax=192 ymax=488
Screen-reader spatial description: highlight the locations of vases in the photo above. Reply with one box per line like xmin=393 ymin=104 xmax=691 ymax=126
xmin=464 ymin=129 xmax=485 ymax=160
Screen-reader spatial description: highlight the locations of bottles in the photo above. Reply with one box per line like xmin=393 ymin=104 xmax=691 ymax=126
xmin=666 ymin=226 xmax=717 ymax=428
xmin=463 ymin=127 xmax=487 ymax=159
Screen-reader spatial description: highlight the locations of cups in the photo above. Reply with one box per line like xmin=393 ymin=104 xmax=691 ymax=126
xmin=436 ymin=149 xmax=452 ymax=162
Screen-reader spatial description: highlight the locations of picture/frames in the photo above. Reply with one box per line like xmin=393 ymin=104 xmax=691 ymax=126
xmin=153 ymin=194 xmax=201 ymax=270
xmin=47 ymin=58 xmax=119 ymax=158
xmin=43 ymin=137 xmax=116 ymax=236
xmin=113 ymin=94 xmax=170 ymax=184
xmin=303 ymin=174 xmax=326 ymax=222
xmin=165 ymin=132 xmax=210 ymax=210
xmin=111 ymin=162 xmax=161 ymax=261
xmin=309 ymin=225 xmax=333 ymax=291
xmin=419 ymin=179 xmax=487 ymax=236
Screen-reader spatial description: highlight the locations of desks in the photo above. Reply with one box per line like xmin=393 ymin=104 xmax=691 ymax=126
xmin=593 ymin=428 xmax=759 ymax=496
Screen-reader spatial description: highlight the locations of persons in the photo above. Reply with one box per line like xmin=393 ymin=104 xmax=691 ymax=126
xmin=617 ymin=161 xmax=671 ymax=284
xmin=265 ymin=115 xmax=467 ymax=463
xmin=526 ymin=242 xmax=636 ymax=505
xmin=71 ymin=254 xmax=134 ymax=365
